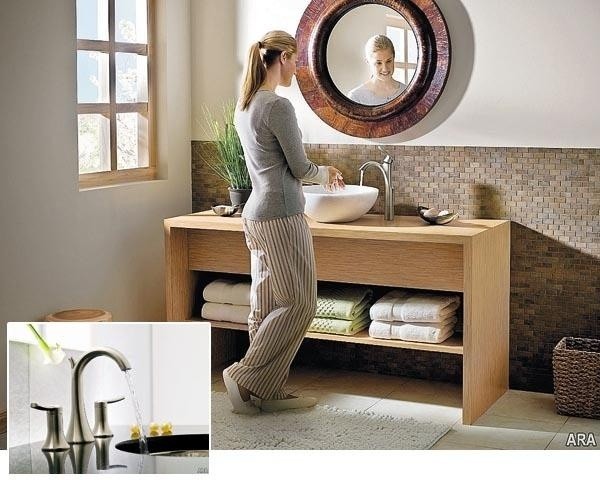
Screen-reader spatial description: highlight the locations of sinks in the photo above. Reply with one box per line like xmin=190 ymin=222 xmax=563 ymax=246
xmin=114 ymin=433 xmax=209 ymax=458
xmin=298 ymin=182 xmax=380 ymax=225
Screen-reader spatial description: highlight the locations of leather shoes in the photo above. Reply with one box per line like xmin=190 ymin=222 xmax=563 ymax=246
xmin=262 ymin=395 xmax=316 ymax=412
xmin=223 ymin=368 xmax=260 ymax=414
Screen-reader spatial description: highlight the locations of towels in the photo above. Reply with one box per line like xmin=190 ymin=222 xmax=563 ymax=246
xmin=369 ymin=320 xmax=456 ymax=344
xmin=312 ymin=287 xmax=373 ymax=321
xmin=201 ymin=303 xmax=251 ymax=324
xmin=202 ymin=278 xmax=251 ymax=306
xmin=369 ymin=289 xmax=460 ymax=322
xmin=307 ymin=310 xmax=369 ymax=336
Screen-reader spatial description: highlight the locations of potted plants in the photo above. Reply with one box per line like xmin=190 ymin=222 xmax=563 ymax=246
xmin=191 ymin=96 xmax=252 ymax=213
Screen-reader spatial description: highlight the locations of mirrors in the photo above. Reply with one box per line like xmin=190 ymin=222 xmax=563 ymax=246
xmin=293 ymin=0 xmax=453 ymax=139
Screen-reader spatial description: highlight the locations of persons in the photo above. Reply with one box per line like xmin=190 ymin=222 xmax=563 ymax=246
xmin=347 ymin=35 xmax=408 ymax=106
xmin=222 ymin=30 xmax=345 ymax=414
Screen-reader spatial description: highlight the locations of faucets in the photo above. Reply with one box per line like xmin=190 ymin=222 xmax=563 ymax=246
xmin=358 ymin=142 xmax=394 ymax=221
xmin=64 ymin=346 xmax=131 ymax=445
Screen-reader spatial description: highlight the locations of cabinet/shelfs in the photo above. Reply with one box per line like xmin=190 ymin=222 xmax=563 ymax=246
xmin=163 ymin=206 xmax=511 ymax=425
